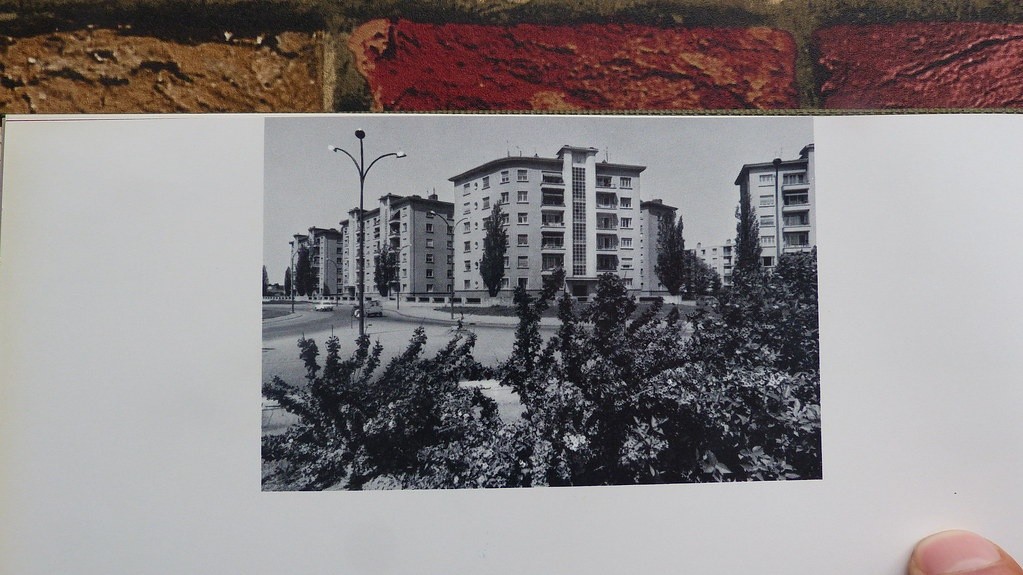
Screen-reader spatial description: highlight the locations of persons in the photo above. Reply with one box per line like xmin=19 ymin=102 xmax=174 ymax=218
xmin=661 ymin=302 xmax=680 ymax=329
xmin=903 ymin=527 xmax=1023 ymax=575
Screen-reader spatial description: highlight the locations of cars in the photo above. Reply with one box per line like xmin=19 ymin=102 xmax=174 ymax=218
xmin=354 ymin=299 xmax=384 ymax=318
xmin=315 ymin=300 xmax=333 ymax=312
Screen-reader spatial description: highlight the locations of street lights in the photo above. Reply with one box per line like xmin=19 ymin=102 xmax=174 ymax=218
xmin=328 ymin=128 xmax=407 ymax=336
xmin=291 ymin=249 xmax=302 ymax=313
xmin=428 ymin=210 xmax=473 ymax=320
xmin=325 ymin=258 xmax=339 ymax=306
